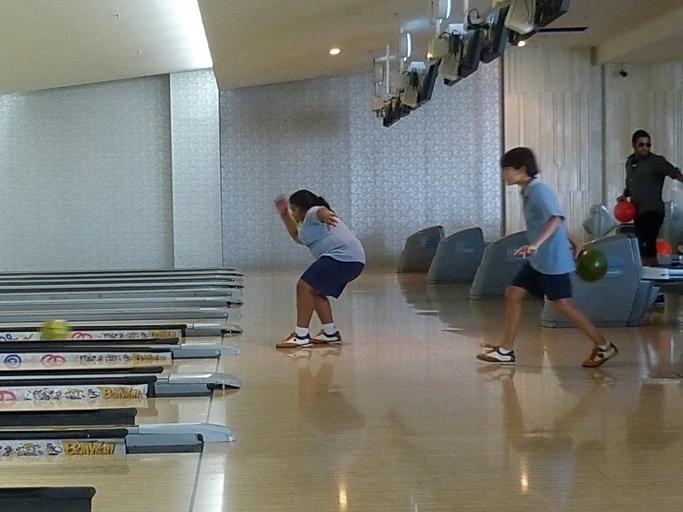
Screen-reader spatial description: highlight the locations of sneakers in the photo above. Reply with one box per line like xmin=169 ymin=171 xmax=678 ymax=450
xmin=581 ymin=336 xmax=619 ymax=367
xmin=476 ymin=343 xmax=515 ymax=363
xmin=276 ymin=332 xmax=311 ymax=348
xmin=310 ymin=331 xmax=342 ymax=347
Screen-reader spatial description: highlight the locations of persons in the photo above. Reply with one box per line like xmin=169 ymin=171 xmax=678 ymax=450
xmin=624 ymin=383 xmax=683 ymax=460
xmin=272 ymin=189 xmax=366 ymax=348
xmin=475 ymin=362 xmax=617 ymax=512
xmin=280 ymin=347 xmax=369 ymax=486
xmin=477 ymin=146 xmax=619 ymax=368
xmin=617 ymin=129 xmax=683 ymax=267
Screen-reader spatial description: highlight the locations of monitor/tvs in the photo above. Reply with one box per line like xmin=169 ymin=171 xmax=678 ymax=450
xmin=382 ymin=57 xmax=442 ymax=128
xmin=444 ymin=0 xmax=571 ymax=87
